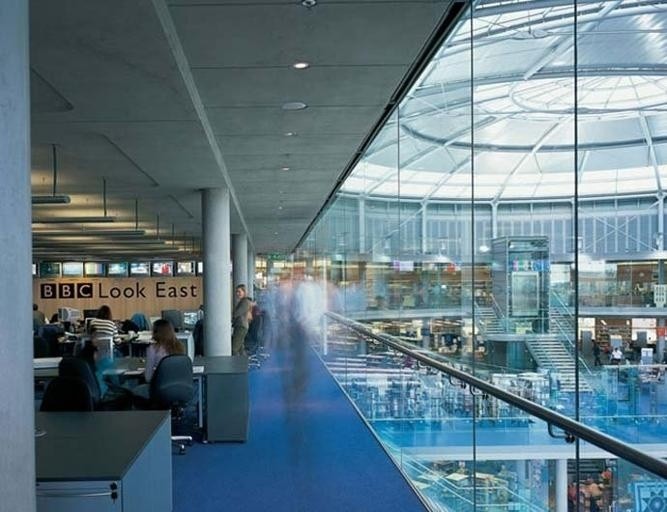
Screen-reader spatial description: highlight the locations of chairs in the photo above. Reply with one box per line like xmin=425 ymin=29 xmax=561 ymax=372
xmin=41 ymin=352 xmax=131 ymax=412
xmin=133 ymin=354 xmax=195 ymax=454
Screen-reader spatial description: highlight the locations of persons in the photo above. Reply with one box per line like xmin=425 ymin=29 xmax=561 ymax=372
xmin=231 ymin=284 xmax=261 ymax=357
xmin=634 ymin=282 xmax=644 ymax=294
xmin=592 ymin=340 xmax=604 ymax=366
xmin=568 ymin=466 xmax=611 ymax=511
xmin=33 ymin=303 xmax=46 ymax=330
xmin=610 ymin=348 xmax=623 ymax=363
xmin=89 ymin=305 xmax=122 ymax=357
xmin=145 ymin=319 xmax=186 ymax=383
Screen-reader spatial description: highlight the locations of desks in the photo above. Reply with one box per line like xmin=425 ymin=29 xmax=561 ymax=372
xmin=34 ymin=357 xmax=249 ymax=442
xmin=65 ymin=332 xmax=194 ymax=361
xmin=35 ymin=410 xmax=172 ymax=511
